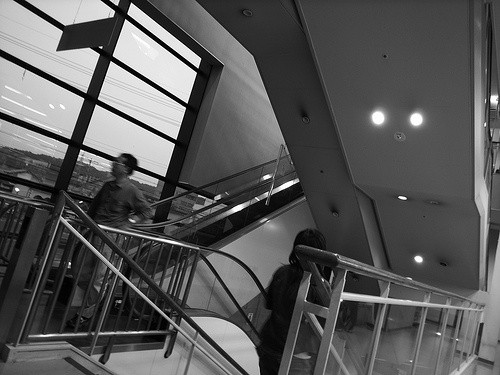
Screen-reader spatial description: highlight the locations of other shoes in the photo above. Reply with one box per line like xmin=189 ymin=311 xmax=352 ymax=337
xmin=66 ymin=313 xmax=92 ymax=327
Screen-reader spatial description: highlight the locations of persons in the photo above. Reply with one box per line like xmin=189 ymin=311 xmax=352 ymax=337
xmin=256 ymin=229 xmax=334 ymax=372
xmin=67 ymin=153 xmax=156 ymax=333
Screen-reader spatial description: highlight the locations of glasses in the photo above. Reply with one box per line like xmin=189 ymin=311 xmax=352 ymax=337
xmin=112 ymin=161 xmax=128 ymax=168
